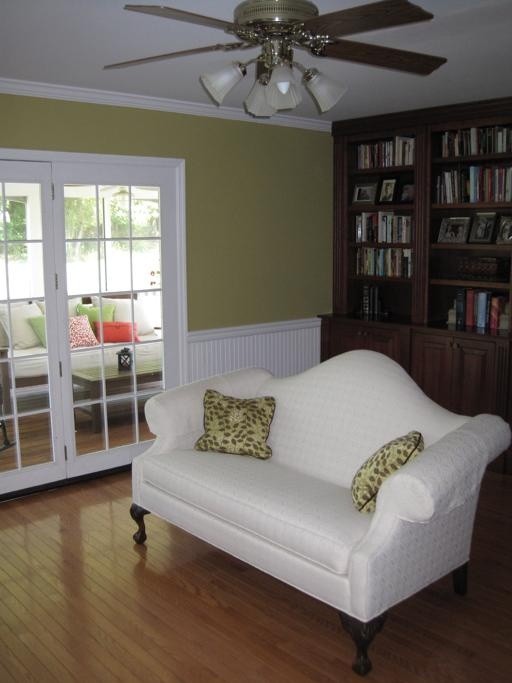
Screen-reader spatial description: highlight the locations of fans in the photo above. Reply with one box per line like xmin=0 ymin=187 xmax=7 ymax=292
xmin=101 ymin=0 xmax=449 ymax=76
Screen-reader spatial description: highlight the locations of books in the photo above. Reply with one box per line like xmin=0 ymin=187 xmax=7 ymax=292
xmin=349 ymin=125 xmax=512 ymax=330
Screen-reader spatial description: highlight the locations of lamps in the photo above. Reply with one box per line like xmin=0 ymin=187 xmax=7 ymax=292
xmin=116 ymin=347 xmax=133 ymax=370
xmin=197 ymin=53 xmax=350 ymax=119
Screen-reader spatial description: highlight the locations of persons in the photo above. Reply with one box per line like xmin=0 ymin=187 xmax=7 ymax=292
xmin=357 ymin=188 xmax=369 ymax=200
xmin=382 ymin=183 xmax=393 ymax=202
xmin=445 ymin=224 xmax=454 ymax=239
xmin=500 ymin=221 xmax=512 ymax=240
xmin=476 ymin=219 xmax=484 ymax=238
xmin=480 ymin=217 xmax=492 ymax=239
xmin=403 ymin=184 xmax=414 ymax=199
xmin=457 ymin=226 xmax=464 ymax=240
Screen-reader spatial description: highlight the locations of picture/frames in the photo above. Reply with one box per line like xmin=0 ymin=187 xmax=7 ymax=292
xmin=377 ymin=178 xmax=396 ymax=205
xmin=467 ymin=211 xmax=496 ymax=244
xmin=353 ymin=184 xmax=376 ymax=205
xmin=399 ymin=183 xmax=416 ymax=204
xmin=495 ymin=214 xmax=512 ymax=244
xmin=435 ymin=216 xmax=469 ymax=245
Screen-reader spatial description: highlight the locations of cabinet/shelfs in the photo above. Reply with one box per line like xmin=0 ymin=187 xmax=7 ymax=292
xmin=423 ymin=96 xmax=512 ymax=325
xmin=411 ymin=327 xmax=511 ymax=474
xmin=317 ymin=97 xmax=423 ymax=323
xmin=318 ymin=313 xmax=411 ymax=375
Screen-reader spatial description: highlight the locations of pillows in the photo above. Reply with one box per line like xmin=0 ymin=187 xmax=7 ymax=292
xmin=195 ymin=389 xmax=275 ymax=461
xmin=5 ymin=300 xmax=146 ymax=350
xmin=350 ymin=432 xmax=423 ymax=517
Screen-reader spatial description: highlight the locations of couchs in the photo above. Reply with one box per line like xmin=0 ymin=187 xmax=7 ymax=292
xmin=130 ymin=349 xmax=511 ymax=675
xmin=0 ymin=294 xmax=162 ymax=419
